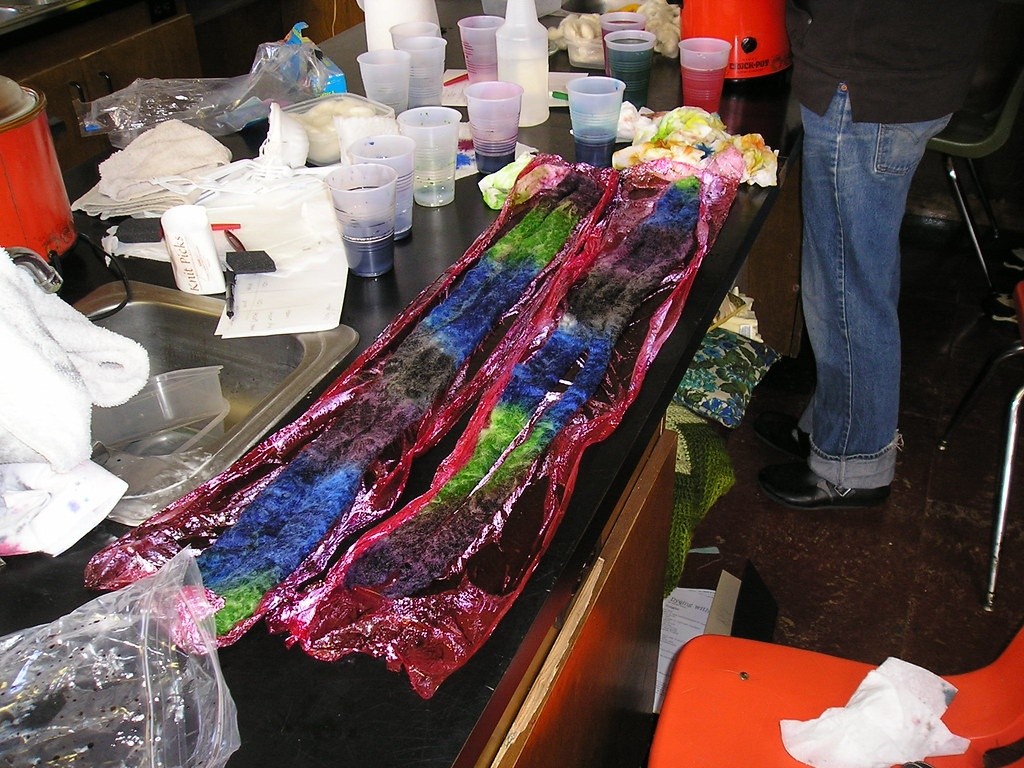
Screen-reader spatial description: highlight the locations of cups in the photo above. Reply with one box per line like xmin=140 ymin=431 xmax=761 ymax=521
xmin=349 ymin=134 xmax=417 ymax=234
xmin=388 ymin=21 xmax=439 ymax=50
xmin=394 ymin=36 xmax=448 ymax=110
xmin=324 ymin=163 xmax=399 ymax=277
xmin=599 ymin=12 xmax=646 ymax=77
xmin=356 ymin=49 xmax=411 ymax=120
xmin=397 ymin=106 xmax=462 ymax=207
xmin=565 ymin=77 xmax=627 ymax=167
xmin=463 ymin=81 xmax=524 ymax=174
xmin=456 ymin=15 xmax=505 ymax=83
xmin=604 ymin=29 xmax=657 ymax=92
xmin=678 ymin=37 xmax=733 ymax=114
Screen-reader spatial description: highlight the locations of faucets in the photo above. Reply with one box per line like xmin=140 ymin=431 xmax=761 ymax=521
xmin=7 ymin=246 xmax=64 ymax=295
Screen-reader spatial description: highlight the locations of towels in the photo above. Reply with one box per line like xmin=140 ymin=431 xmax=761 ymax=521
xmin=72 ymin=118 xmax=233 ymax=220
xmin=0 ymin=245 xmax=150 ymax=476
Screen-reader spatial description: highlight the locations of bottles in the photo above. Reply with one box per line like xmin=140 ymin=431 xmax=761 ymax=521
xmin=495 ymin=0 xmax=550 ymax=128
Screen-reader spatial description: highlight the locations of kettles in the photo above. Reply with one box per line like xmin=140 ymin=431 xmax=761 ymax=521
xmin=664 ymin=0 xmax=795 ymax=86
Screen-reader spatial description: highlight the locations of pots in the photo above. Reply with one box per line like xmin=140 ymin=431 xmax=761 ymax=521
xmin=0 ymin=74 xmax=132 ymax=321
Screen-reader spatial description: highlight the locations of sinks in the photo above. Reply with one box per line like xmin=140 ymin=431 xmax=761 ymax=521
xmin=71 ymin=278 xmax=360 ymax=527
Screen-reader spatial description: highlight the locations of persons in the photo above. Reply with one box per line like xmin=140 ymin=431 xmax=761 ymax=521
xmin=754 ymin=2 xmax=993 ymax=511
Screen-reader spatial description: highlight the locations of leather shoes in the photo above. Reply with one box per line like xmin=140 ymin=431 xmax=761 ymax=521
xmin=758 ymin=462 xmax=891 ymax=510
xmin=754 ymin=410 xmax=811 ymax=461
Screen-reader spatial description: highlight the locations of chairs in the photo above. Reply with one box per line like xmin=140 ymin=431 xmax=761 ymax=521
xmin=652 ymin=623 xmax=1024 ymax=768
xmin=926 ymin=80 xmax=1024 ymax=611
